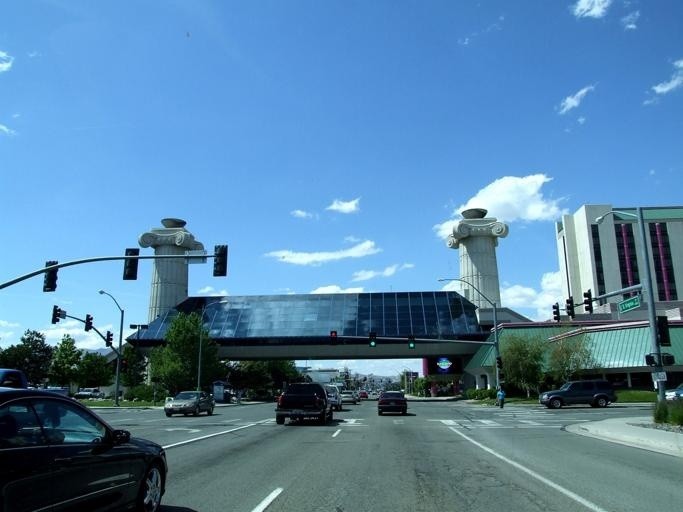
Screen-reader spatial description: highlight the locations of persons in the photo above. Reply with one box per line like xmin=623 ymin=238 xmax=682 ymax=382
xmin=496 ymin=387 xmax=506 ymax=409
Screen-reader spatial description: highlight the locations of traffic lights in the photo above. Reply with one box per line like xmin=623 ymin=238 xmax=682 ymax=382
xmin=105 ymin=330 xmax=114 ymax=348
xmin=496 ymin=356 xmax=502 ymax=370
xmin=330 ymin=330 xmax=338 ymax=346
xmin=408 ymin=333 xmax=416 ymax=349
xmin=658 ymin=316 xmax=671 ymax=346
xmin=121 ymin=358 xmax=127 ymax=373
xmin=646 ymin=355 xmax=654 ymax=365
xmin=84 ymin=313 xmax=93 ymax=333
xmin=369 ymin=332 xmax=377 ymax=347
xmin=582 ymin=288 xmax=594 ymax=314
xmin=552 ymin=302 xmax=561 ymax=322
xmin=565 ymin=296 xmax=575 ymax=319
xmin=661 ymin=353 xmax=676 ymax=366
xmin=52 ymin=305 xmax=61 ymax=326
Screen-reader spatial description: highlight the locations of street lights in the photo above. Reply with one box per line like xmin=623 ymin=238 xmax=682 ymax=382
xmin=98 ymin=289 xmax=126 ymax=406
xmin=197 ymin=300 xmax=229 ymax=392
xmin=437 ymin=277 xmax=502 ymax=393
xmin=128 ymin=322 xmax=149 ymax=350
xmin=594 ymin=206 xmax=664 ymax=401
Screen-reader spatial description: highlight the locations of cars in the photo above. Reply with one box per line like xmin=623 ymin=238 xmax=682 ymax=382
xmin=322 ymin=383 xmax=383 ymax=412
xmin=376 ymin=388 xmax=409 ymax=417
xmin=0 ymin=368 xmax=219 ymax=512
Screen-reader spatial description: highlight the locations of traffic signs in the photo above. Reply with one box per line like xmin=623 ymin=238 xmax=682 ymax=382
xmin=651 ymin=372 xmax=666 ymax=381
xmin=617 ymin=295 xmax=642 ymax=314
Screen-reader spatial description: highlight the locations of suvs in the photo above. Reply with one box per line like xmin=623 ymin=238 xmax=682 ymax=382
xmin=656 ymin=384 xmax=683 ymax=402
xmin=274 ymin=381 xmax=335 ymax=426
xmin=537 ymin=379 xmax=620 ymax=409
